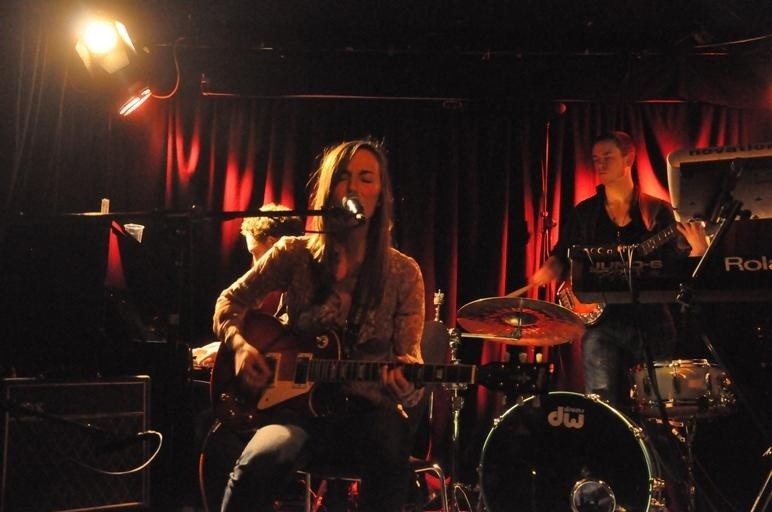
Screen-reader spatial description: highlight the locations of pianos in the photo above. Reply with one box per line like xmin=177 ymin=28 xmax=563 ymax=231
xmin=571 ymin=256 xmax=771 ymax=302
xmin=666 ymin=142 xmax=772 ymax=222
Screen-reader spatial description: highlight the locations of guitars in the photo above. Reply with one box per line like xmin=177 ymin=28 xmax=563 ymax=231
xmin=556 ymin=220 xmax=679 ymax=330
xmin=211 ymin=313 xmax=556 ymax=436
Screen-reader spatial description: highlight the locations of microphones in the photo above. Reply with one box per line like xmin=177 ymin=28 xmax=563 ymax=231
xmin=525 ymin=102 xmax=568 ymax=116
xmin=711 ymin=157 xmax=746 ymax=225
xmin=342 ymin=193 xmax=366 ymax=222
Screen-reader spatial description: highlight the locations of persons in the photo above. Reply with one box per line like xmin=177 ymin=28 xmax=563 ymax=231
xmin=241 ymin=203 xmax=302 ymax=313
xmin=532 ymin=131 xmax=710 ymax=412
xmin=213 ymin=140 xmax=424 ymax=511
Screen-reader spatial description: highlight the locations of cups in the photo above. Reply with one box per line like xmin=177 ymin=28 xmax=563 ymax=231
xmin=124 ymin=224 xmax=145 ymax=243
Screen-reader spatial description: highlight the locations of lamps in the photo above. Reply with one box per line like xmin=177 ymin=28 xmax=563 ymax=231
xmin=119 ymin=86 xmax=159 ymax=127
xmin=75 ymin=13 xmax=136 ymax=79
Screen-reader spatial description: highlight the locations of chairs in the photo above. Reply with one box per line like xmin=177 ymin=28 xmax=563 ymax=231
xmin=296 ymin=321 xmax=458 ymax=512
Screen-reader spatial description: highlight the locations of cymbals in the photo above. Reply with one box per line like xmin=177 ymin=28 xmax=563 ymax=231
xmin=457 ymin=296 xmax=585 ymax=347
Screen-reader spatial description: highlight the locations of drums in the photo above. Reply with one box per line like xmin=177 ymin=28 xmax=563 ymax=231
xmin=634 ymin=359 xmax=738 ymax=422
xmin=477 ymin=392 xmax=665 ymax=511
